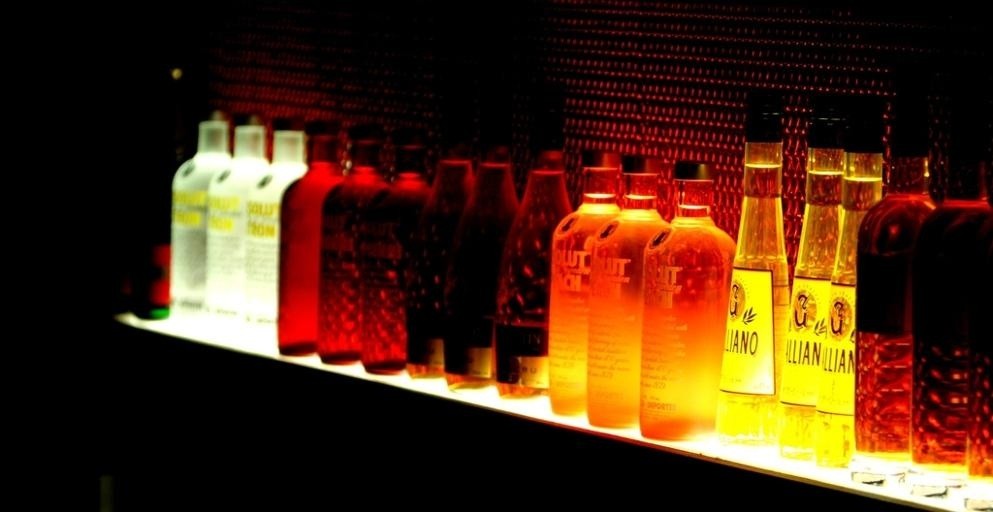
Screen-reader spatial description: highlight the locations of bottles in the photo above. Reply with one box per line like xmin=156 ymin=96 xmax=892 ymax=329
xmin=242 ymin=131 xmax=310 ymax=311
xmin=587 ymin=175 xmax=671 ymax=431
xmin=364 ymin=146 xmax=434 ymax=374
xmin=208 ymin=127 xmax=275 ymax=320
xmin=639 ymin=179 xmax=738 ymax=439
xmin=912 ymin=124 xmax=992 ymax=508
xmin=784 ymin=126 xmax=847 ymax=454
xmin=716 ymin=113 xmax=796 ymax=444
xmin=820 ymin=124 xmax=896 ymax=464
xmin=175 ymin=118 xmax=231 ymax=315
xmin=499 ymin=167 xmax=570 ymax=397
xmin=408 ymin=158 xmax=478 ymax=378
xmin=280 ymin=135 xmax=349 ymax=353
xmin=444 ymin=163 xmax=527 ymax=389
xmin=546 ymin=167 xmax=626 ymax=414
xmin=320 ymin=141 xmax=391 ymax=363
xmin=855 ymin=124 xmax=940 ymax=479
